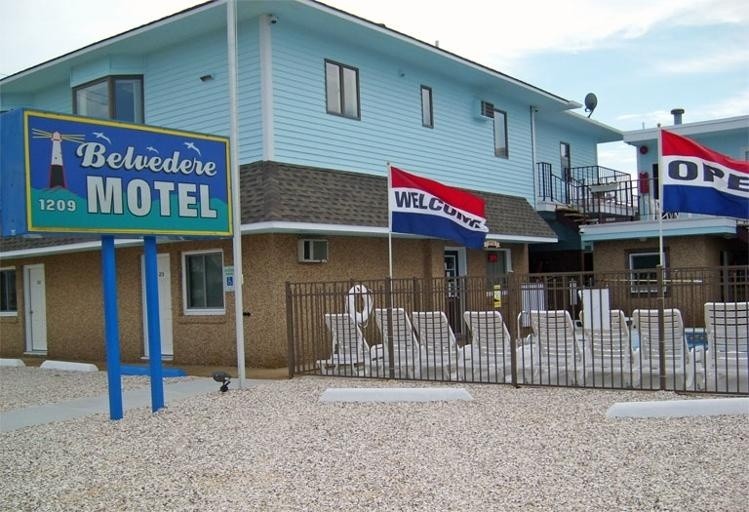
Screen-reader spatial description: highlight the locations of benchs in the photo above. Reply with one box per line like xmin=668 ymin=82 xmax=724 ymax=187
xmin=657 ymin=127 xmax=749 ymax=220
xmin=389 ymin=164 xmax=489 ymax=251
